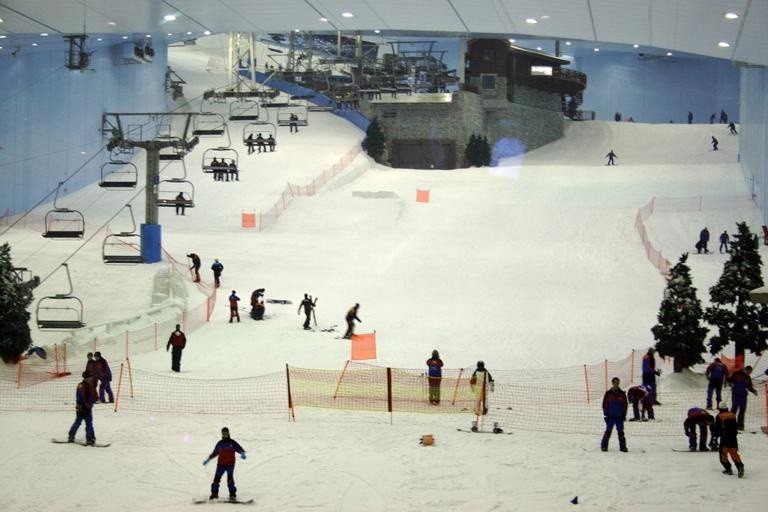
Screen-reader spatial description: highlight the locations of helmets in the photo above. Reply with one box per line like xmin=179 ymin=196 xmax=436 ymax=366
xmin=432 ymin=350 xmax=438 ymax=355
xmin=477 ymin=361 xmax=484 ymax=368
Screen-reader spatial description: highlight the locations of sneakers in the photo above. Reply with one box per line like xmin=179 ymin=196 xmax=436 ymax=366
xmin=690 ymin=447 xmax=696 ymax=452
xmin=629 ymin=417 xmax=640 ymax=421
xmin=602 ymin=447 xmax=608 ymax=451
xmin=87 ymin=440 xmax=95 ymax=445
xmin=229 ymin=493 xmax=236 ymax=499
xmin=620 ymin=447 xmax=627 ymax=452
xmin=471 ymin=426 xmax=477 ymax=431
xmin=700 ymin=447 xmax=709 ymax=451
xmin=493 ymin=428 xmax=502 ymax=432
xmin=722 ymin=470 xmax=732 ymax=475
xmin=649 ymin=415 xmax=655 ymax=420
xmin=69 ymin=437 xmax=74 ymax=441
xmin=209 ymin=494 xmax=218 ymax=499
xmin=738 ymin=464 xmax=744 ymax=477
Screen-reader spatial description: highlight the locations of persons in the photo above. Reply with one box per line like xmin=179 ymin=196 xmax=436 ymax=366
xmin=470 ymin=360 xmax=494 ymax=414
xmin=247 ymin=133 xmax=275 ymax=154
xmin=211 ymin=258 xmax=224 ymax=287
xmin=695 ymin=226 xmax=730 ymax=255
xmin=606 ymin=149 xmax=617 ymax=165
xmin=67 ymin=351 xmax=113 ymax=447
xmin=166 ymin=324 xmax=186 ymax=372
xmin=175 ymin=192 xmax=185 ymax=215
xmin=229 ymin=287 xmax=266 ymax=322
xmin=426 ymin=350 xmax=444 ymax=407
xmin=211 ymin=157 xmax=240 ymax=182
xmin=711 ymin=136 xmax=718 ymax=150
xmin=343 ymin=303 xmax=362 ymax=339
xmin=186 ymin=252 xmax=201 ymax=282
xmin=598 ymin=345 xmax=757 ymax=502
xmin=203 ymin=427 xmax=247 ymax=499
xmin=687 ymin=108 xmax=738 ymax=134
xmin=298 ymin=293 xmax=316 ymax=330
xmin=289 ymin=113 xmax=298 ymax=133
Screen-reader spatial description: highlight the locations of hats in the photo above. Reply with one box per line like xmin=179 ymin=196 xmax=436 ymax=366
xmin=719 ymin=401 xmax=728 ymax=409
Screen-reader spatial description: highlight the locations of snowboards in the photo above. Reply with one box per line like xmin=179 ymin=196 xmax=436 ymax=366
xmin=52 ymin=435 xmax=112 ymax=448
xmin=457 ymin=428 xmax=514 ymax=435
xmin=267 ymin=299 xmax=292 ymax=304
xmin=673 ymin=448 xmax=718 ymax=452
xmin=191 ymin=492 xmax=255 ymax=504
